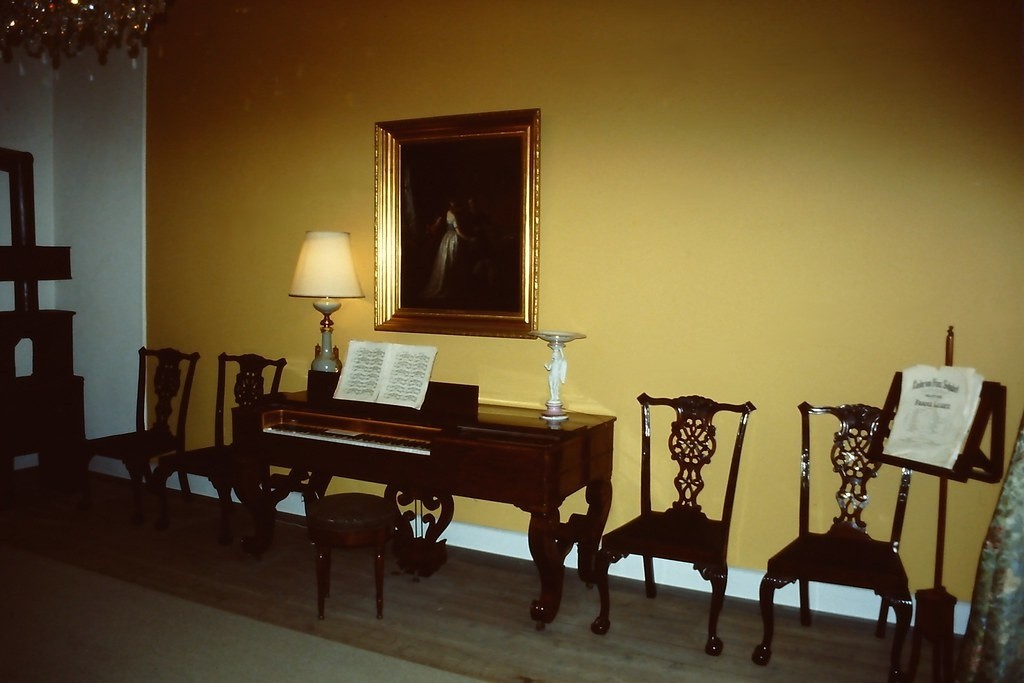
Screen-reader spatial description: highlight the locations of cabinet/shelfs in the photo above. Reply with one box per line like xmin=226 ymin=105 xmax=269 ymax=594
xmin=0 ymin=147 xmax=86 ymax=510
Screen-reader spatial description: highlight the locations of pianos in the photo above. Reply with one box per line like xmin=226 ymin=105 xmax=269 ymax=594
xmin=245 ymin=393 xmax=617 ymax=633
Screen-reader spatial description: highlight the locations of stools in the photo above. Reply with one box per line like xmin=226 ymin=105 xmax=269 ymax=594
xmin=306 ymin=492 xmax=399 ymax=620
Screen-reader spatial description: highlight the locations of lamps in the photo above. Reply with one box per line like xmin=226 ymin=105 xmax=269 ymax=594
xmin=289 ymin=231 xmax=365 ymax=372
xmin=0 ymin=0 xmax=167 ymax=69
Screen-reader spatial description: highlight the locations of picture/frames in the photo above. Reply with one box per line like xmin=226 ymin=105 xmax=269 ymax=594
xmin=373 ymin=106 xmax=541 ymax=341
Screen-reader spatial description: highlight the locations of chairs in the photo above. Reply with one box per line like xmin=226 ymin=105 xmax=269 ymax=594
xmin=590 ymin=392 xmax=756 ymax=656
xmin=157 ymin=352 xmax=287 ymax=548
xmin=77 ymin=345 xmax=201 ymax=521
xmin=752 ymin=401 xmax=913 ymax=683
